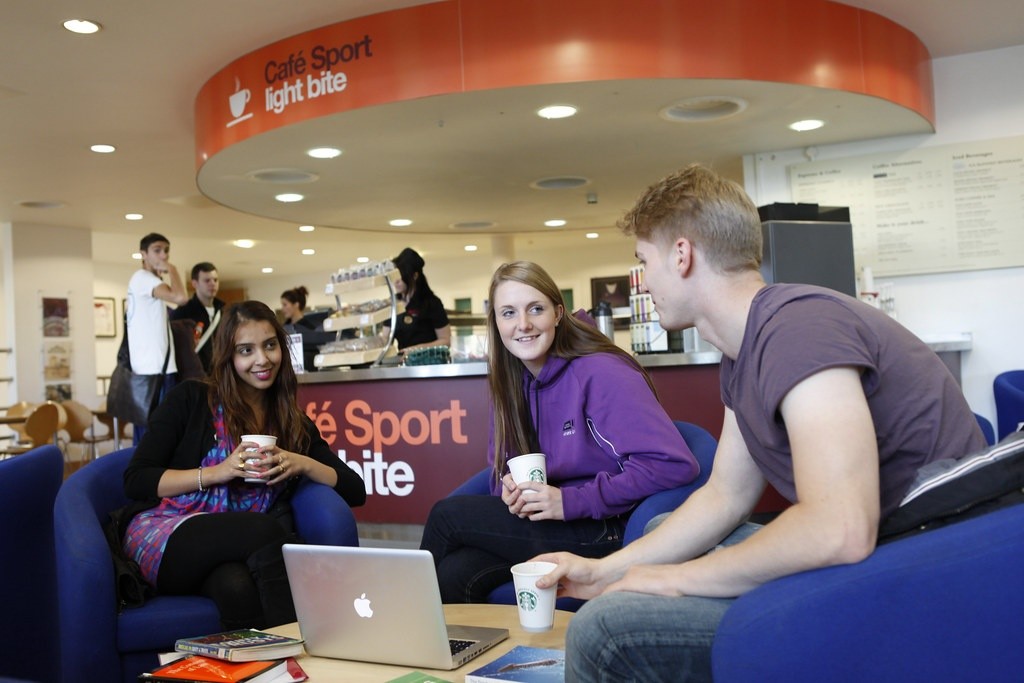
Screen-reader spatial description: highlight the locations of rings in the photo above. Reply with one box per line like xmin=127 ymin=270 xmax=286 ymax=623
xmin=278 ymin=454 xmax=282 ymax=464
xmin=239 ymin=452 xmax=243 ymax=459
xmin=278 ymin=464 xmax=285 ymax=472
xmin=238 ymin=462 xmax=245 ymax=471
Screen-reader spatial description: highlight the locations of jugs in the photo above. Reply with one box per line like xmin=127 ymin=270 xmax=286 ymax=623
xmin=592 ymin=300 xmax=614 ymax=344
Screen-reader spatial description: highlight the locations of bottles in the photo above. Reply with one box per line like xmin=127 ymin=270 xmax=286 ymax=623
xmin=192 ymin=321 xmax=204 ymax=344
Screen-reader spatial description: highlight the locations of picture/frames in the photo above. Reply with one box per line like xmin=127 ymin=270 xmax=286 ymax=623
xmin=93 ymin=297 xmax=117 ymax=338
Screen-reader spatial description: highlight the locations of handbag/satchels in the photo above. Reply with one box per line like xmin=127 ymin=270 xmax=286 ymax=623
xmin=107 ymin=364 xmax=164 ymax=425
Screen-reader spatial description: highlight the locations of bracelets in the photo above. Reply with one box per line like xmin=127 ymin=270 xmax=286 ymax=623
xmin=199 ymin=466 xmax=208 ymax=492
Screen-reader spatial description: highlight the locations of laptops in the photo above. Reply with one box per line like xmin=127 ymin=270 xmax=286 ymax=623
xmin=281 ymin=543 xmax=509 ymax=671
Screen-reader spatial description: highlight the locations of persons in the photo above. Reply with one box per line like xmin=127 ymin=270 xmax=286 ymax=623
xmin=114 ymin=301 xmax=367 ymax=631
xmin=525 ymin=164 xmax=988 ymax=683
xmin=170 ymin=262 xmax=226 ymax=373
xmin=108 ymin=312 xmax=166 ymax=445
xmin=126 ymin=232 xmax=184 ymax=420
xmin=280 ymin=285 xmax=321 ymax=373
xmin=382 ymin=248 xmax=450 ymax=362
xmin=419 ymin=260 xmax=700 ymax=606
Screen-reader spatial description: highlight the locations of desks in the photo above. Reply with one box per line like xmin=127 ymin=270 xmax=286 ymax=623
xmin=262 ymin=604 xmax=576 ymax=683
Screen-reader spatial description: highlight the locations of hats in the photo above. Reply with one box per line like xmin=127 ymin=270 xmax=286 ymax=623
xmin=396 ymin=248 xmax=425 ymax=268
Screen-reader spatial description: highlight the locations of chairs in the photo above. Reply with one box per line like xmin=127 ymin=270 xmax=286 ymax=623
xmin=0 ymin=396 xmax=135 ymax=485
xmin=0 ymin=445 xmax=65 ymax=683
xmin=994 ymin=369 xmax=1024 ymax=445
xmin=54 ymin=446 xmax=360 ymax=683
xmin=447 ymin=421 xmax=717 ymax=613
xmin=711 ymin=501 xmax=1024 ymax=683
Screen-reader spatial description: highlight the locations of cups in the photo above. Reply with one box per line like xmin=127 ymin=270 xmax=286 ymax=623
xmin=507 ymin=452 xmax=547 ymax=495
xmin=510 ymin=562 xmax=558 ymax=632
xmin=241 ymin=434 xmax=278 ymax=484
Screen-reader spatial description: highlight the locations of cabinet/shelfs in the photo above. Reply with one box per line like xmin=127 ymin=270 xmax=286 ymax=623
xmin=313 ymin=269 xmax=406 ymax=372
